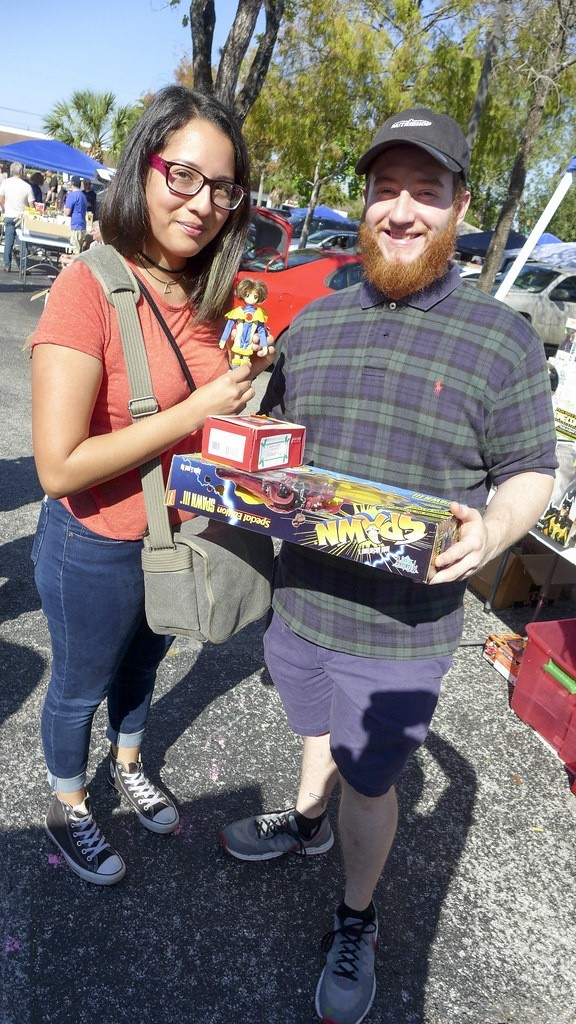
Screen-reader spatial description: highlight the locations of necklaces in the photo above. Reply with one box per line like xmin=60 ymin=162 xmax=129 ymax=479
xmin=135 ymin=250 xmax=186 ymax=293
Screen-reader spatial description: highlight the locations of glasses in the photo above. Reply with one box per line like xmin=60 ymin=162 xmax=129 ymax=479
xmin=151 ymin=153 xmax=248 ymax=214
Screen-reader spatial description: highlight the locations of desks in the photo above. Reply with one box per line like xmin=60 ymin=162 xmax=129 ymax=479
xmin=483 ymin=527 xmax=576 ymax=622
xmin=15 ymin=229 xmax=76 ymax=285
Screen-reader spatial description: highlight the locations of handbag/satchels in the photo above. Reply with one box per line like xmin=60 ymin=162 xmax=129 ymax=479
xmin=141 ymin=507 xmax=275 ymax=643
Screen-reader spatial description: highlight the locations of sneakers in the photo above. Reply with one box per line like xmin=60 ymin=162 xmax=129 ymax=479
xmin=44 ymin=793 xmax=125 ymax=888
xmin=107 ymin=745 xmax=181 ymax=835
xmin=221 ymin=810 xmax=336 ymax=861
xmin=315 ymin=899 xmax=379 ymax=1022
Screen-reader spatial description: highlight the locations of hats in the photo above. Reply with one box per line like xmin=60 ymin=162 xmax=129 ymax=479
xmin=70 ymin=175 xmax=81 ymax=186
xmin=354 ymin=108 xmax=471 ymax=185
xmin=83 ymin=178 xmax=91 ymax=184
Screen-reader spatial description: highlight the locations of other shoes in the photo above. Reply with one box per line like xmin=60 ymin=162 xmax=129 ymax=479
xmin=3 ymin=268 xmax=11 ymax=273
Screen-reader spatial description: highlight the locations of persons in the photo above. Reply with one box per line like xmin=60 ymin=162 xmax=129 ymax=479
xmin=82 ymin=178 xmax=96 ymax=212
xmin=24 ymin=172 xmax=44 ymax=202
xmin=64 ymin=176 xmax=87 ymax=254
xmin=30 ymin=85 xmax=275 ymax=885
xmin=220 ymin=108 xmax=559 ymax=1024
xmin=44 ymin=170 xmax=58 ymax=201
xmin=218 ymin=277 xmax=269 ymax=369
xmin=60 ymin=221 xmax=105 ymax=268
xmin=0 ymin=160 xmax=36 ymax=273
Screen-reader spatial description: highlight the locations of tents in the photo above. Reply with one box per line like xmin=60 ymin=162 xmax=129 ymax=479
xmin=0 ymin=136 xmax=105 ymax=181
xmin=289 ymin=205 xmax=351 ymax=224
xmin=455 ymin=229 xmax=563 ymax=257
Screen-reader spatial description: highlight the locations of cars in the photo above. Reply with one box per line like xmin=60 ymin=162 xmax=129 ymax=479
xmin=245 ymin=215 xmax=361 ymax=259
xmin=459 ymin=260 xmax=576 ymax=360
xmin=230 ymin=206 xmax=366 ymax=373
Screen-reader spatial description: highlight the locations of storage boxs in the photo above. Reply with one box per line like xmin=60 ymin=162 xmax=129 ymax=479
xmin=163 ymin=452 xmax=467 ymax=585
xmin=509 ymin=618 xmax=576 ymax=777
xmin=469 ymin=533 xmax=576 ymax=610
xmin=200 ymin=414 xmax=306 ymax=473
xmin=483 ymin=633 xmax=526 ymax=687
xmin=15 ymin=216 xmax=71 ymax=242
